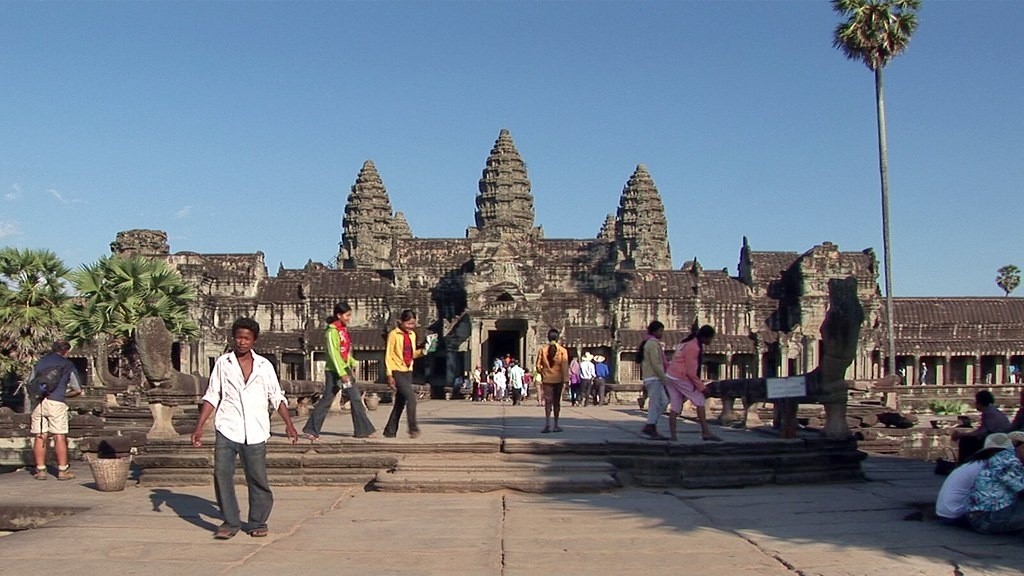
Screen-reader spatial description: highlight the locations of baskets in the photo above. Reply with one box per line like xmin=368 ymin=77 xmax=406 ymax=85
xmin=86 ymin=453 xmax=132 ymax=492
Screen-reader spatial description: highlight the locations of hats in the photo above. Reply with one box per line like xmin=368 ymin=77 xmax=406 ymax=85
xmin=582 ymin=352 xmax=594 ymax=361
xmin=594 ymin=355 xmax=605 ymax=362
xmin=979 ymin=433 xmax=1014 ymax=453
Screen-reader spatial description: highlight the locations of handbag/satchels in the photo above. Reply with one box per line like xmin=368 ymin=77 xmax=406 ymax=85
xmin=934 ymin=445 xmax=959 ymax=477
xmin=25 ymin=365 xmax=65 ymax=406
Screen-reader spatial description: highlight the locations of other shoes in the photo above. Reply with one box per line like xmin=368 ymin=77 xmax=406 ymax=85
xmin=642 ymin=423 xmax=664 ymax=437
xmin=57 ymin=464 xmax=75 ymax=480
xmin=214 ymin=528 xmax=240 ymax=539
xmin=553 ymin=427 xmax=565 ymax=432
xmin=34 ymin=467 xmax=48 ymax=480
xmin=702 ymin=432 xmax=729 ymax=443
xmin=252 ymin=531 xmax=268 ymax=536
xmin=542 ymin=425 xmax=551 ymax=433
xmin=357 ymin=433 xmax=376 ymax=437
xmin=304 ymin=432 xmax=319 ymax=440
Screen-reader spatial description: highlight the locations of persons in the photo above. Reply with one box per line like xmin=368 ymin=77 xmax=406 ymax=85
xmin=536 ymin=329 xmax=570 ymax=433
xmin=472 ymin=353 xmax=610 ymax=407
xmin=936 ymin=390 xmax=1024 ymax=536
xmin=383 ymin=309 xmax=428 ymax=438
xmin=302 ymin=303 xmax=376 ymax=440
xmin=26 ymin=343 xmax=81 ymax=480
xmin=635 ymin=321 xmax=724 ymax=442
xmin=191 ymin=316 xmax=298 ymax=540
